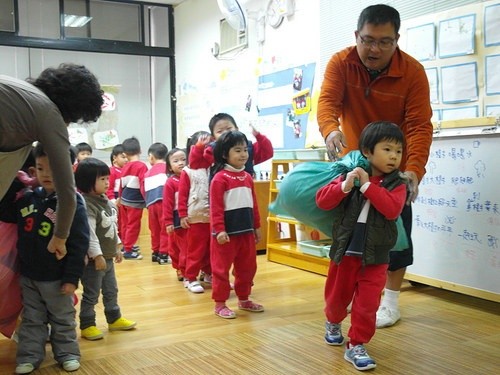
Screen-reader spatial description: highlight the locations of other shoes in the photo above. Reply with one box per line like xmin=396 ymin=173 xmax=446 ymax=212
xmin=188 ymin=281 xmax=205 ymax=293
xmin=152 ymin=252 xmax=159 ymax=262
xmin=121 ymin=245 xmax=141 ymax=252
xmin=214 ymin=303 xmax=236 ymax=319
xmin=203 ymin=274 xmax=212 ymax=283
xmin=124 ymin=252 xmax=143 ymax=260
xmin=157 ymin=253 xmax=173 ymax=264
xmin=15 ymin=362 xmax=34 ymax=373
xmin=376 ymin=306 xmax=400 ymax=327
xmin=237 ymin=300 xmax=265 ymax=313
xmin=63 ymin=360 xmax=81 ymax=372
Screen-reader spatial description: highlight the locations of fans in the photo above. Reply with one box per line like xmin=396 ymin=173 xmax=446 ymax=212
xmin=217 ymin=0 xmax=264 ymax=43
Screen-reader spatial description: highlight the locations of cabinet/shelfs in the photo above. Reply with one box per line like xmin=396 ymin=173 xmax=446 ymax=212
xmin=266 ymin=159 xmax=338 ymax=278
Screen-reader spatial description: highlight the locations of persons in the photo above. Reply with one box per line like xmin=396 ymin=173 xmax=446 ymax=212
xmin=0 ymin=139 xmax=91 ymax=373
xmin=316 ymin=121 xmax=410 ymax=370
xmin=144 ymin=143 xmax=172 ymax=264
xmin=210 ymin=130 xmax=265 ymax=319
xmin=163 ymin=112 xmax=273 ymax=294
xmin=75 ymin=137 xmax=148 ymax=260
xmin=0 ymin=63 xmax=105 ymax=260
xmin=317 ymin=4 xmax=433 ymax=328
xmin=75 ymin=157 xmax=137 ymax=340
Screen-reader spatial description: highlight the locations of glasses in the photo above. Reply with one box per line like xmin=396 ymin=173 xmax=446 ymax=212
xmin=359 ymin=34 xmax=396 ymax=49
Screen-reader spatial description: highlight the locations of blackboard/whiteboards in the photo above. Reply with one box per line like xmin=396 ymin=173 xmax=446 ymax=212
xmin=404 ymin=138 xmax=500 ymax=304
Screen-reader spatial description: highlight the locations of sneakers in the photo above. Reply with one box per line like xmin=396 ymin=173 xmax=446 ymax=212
xmin=108 ymin=317 xmax=136 ymax=331
xmin=325 ymin=319 xmax=345 ymax=346
xmin=81 ymin=325 xmax=102 ymax=339
xmin=344 ymin=341 xmax=377 ymax=370
